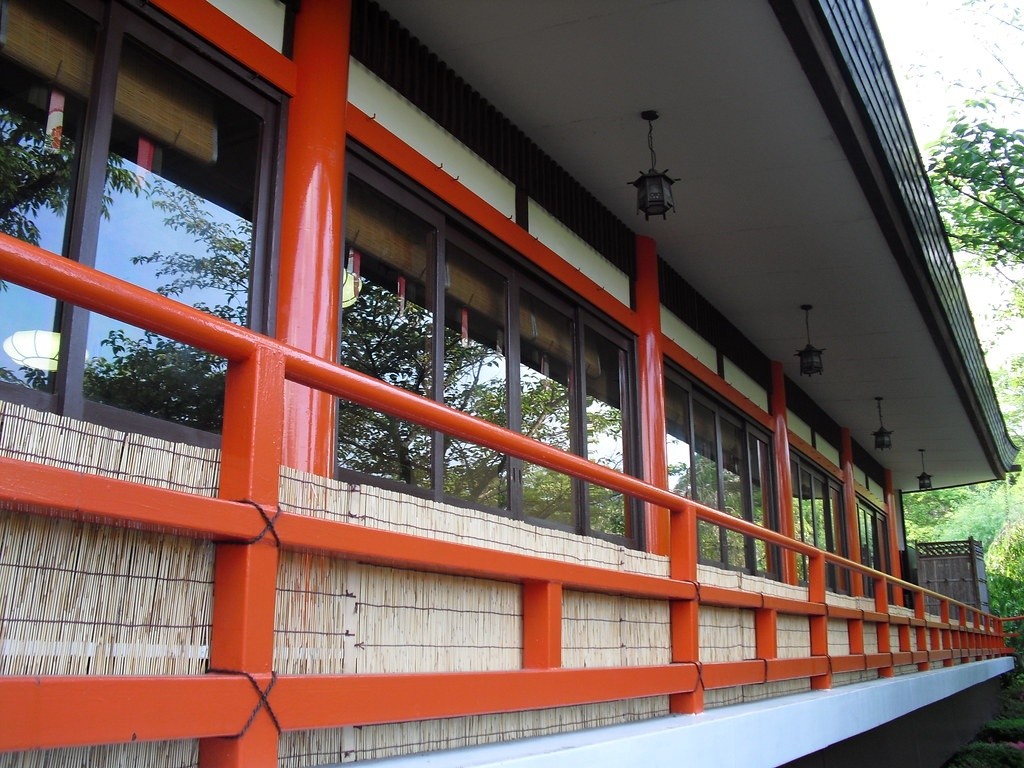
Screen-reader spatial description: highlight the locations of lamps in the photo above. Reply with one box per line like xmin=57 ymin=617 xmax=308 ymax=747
xmin=626 ymin=110 xmax=683 ymax=222
xmin=793 ymin=304 xmax=828 ymax=378
xmin=2 ymin=330 xmax=91 ymax=373
xmin=871 ymin=397 xmax=894 ymax=452
xmin=342 ymin=266 xmax=362 ymax=308
xmin=915 ymin=448 xmax=933 ymax=491
